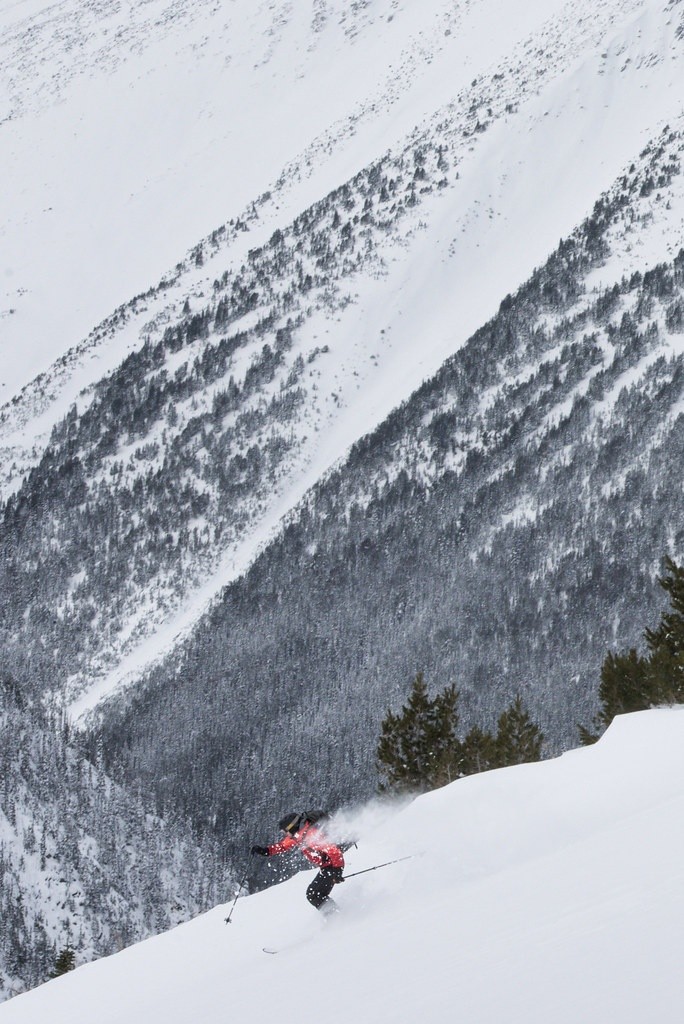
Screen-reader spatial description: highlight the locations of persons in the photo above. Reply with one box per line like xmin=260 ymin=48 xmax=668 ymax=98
xmin=252 ymin=812 xmax=345 ymax=908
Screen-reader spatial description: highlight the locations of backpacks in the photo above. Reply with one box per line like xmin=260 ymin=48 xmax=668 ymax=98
xmin=304 ymin=808 xmax=360 ymax=855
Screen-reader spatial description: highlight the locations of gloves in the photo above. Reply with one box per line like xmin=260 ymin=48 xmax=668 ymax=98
xmin=326 ymin=873 xmax=343 ymax=883
xmin=251 ymin=845 xmax=265 ymax=855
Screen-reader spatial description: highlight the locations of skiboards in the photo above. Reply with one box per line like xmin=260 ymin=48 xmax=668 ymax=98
xmin=264 ymin=946 xmax=287 ymax=955
